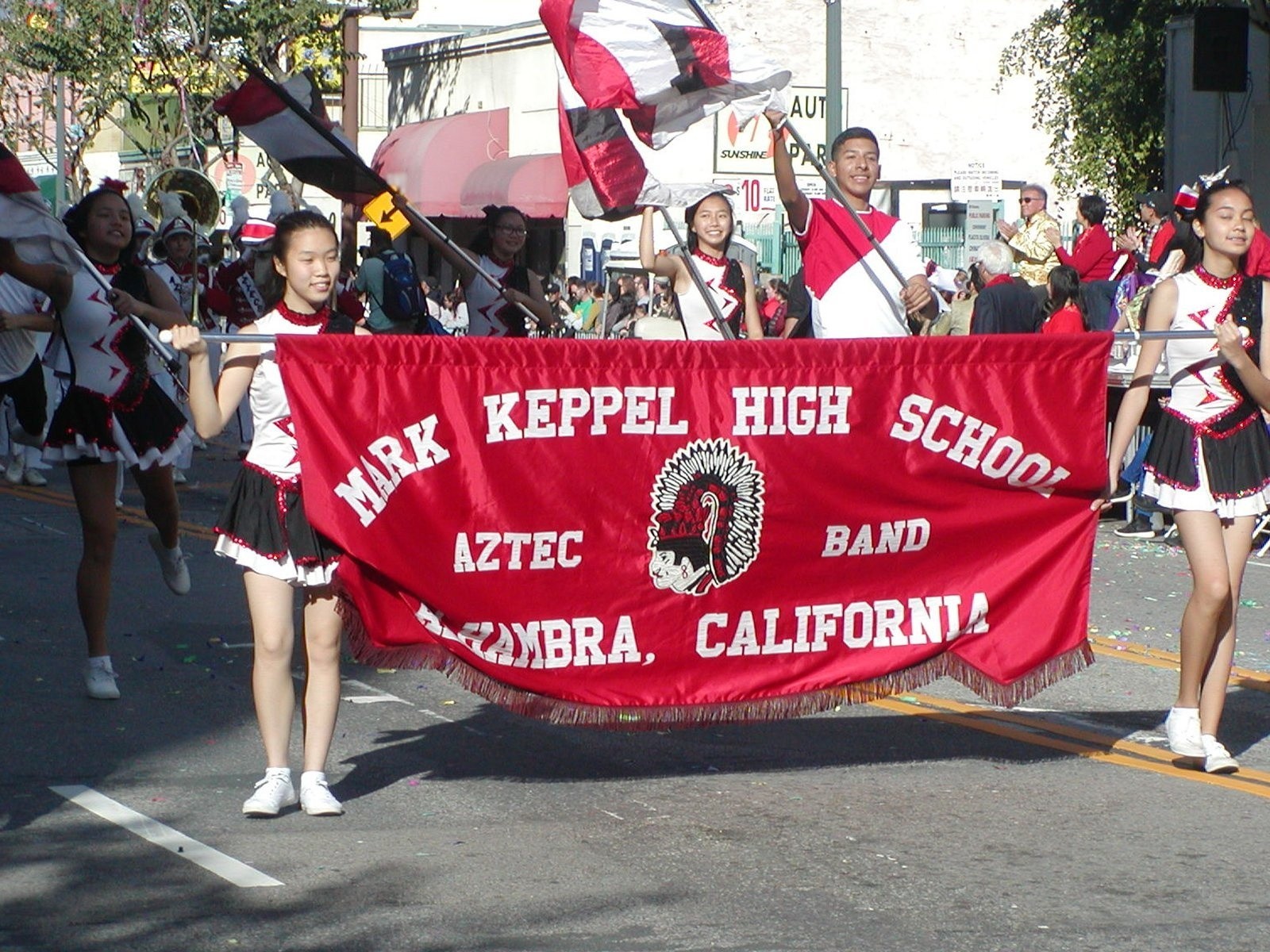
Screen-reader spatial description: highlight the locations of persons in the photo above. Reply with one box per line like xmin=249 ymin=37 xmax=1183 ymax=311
xmin=638 ymin=188 xmax=764 ymax=341
xmin=415 ymin=269 xmax=684 ymax=341
xmin=391 ymin=192 xmax=554 ymax=337
xmin=759 ymin=104 xmax=951 ymax=337
xmin=921 ymin=182 xmax=1187 ymax=538
xmin=0 ymin=207 xmax=472 ymax=486
xmin=1091 ymin=165 xmax=1270 ymax=773
xmin=169 ymin=208 xmax=377 ymax=818
xmin=0 ymin=175 xmax=192 ymax=701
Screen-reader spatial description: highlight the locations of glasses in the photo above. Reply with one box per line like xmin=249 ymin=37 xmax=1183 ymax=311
xmin=493 ymin=225 xmax=527 ymax=236
xmin=1139 ymin=203 xmax=1149 ymax=209
xmin=1019 ymin=197 xmax=1042 ymax=203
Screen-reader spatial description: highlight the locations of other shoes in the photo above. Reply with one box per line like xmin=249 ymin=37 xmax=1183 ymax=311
xmin=173 ymin=469 xmax=186 ymax=483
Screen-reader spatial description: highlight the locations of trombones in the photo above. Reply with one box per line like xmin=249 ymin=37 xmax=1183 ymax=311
xmin=188 ymin=219 xmax=203 ymax=329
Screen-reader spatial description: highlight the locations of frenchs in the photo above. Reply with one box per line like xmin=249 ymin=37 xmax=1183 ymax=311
xmin=143 ymin=167 xmax=221 ymax=237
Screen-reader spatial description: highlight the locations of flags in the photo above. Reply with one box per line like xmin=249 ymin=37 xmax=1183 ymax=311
xmin=553 ymin=29 xmax=671 ymax=223
xmin=1 ymin=142 xmax=87 ymax=268
xmin=536 ymin=0 xmax=794 ymax=153
xmin=212 ymin=55 xmax=397 ymax=203
xmin=268 ymin=332 xmax=1096 ymax=736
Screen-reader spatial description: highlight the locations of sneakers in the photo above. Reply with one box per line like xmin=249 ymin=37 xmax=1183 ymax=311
xmin=1164 ymin=708 xmax=1206 ymax=757
xmin=6 ymin=461 xmax=25 ymax=484
xmin=1113 ymin=520 xmax=1155 ymax=538
xmin=1137 ymin=529 xmax=1168 ymax=542
xmin=242 ymin=771 xmax=299 ymax=815
xmin=81 ymin=660 xmax=121 ymax=699
xmin=149 ymin=532 xmax=191 ymax=595
xmin=1204 ymin=741 xmax=1239 ymax=774
xmin=23 ymin=469 xmax=48 ymax=486
xmin=300 ymin=778 xmax=343 ymax=815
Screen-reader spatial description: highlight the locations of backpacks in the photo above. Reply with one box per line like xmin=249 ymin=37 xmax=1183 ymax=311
xmin=375 ymin=250 xmax=426 ymax=322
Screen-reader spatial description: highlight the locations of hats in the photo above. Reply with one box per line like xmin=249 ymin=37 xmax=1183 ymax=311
xmin=158 ymin=190 xmax=194 ymax=246
xmin=127 ymin=193 xmax=156 ymax=236
xmin=240 ymin=217 xmax=277 ymax=246
xmin=1135 ymin=190 xmax=1169 ymax=216
xmin=228 ymin=195 xmax=251 ymax=245
xmin=266 ymin=190 xmax=293 ymax=225
